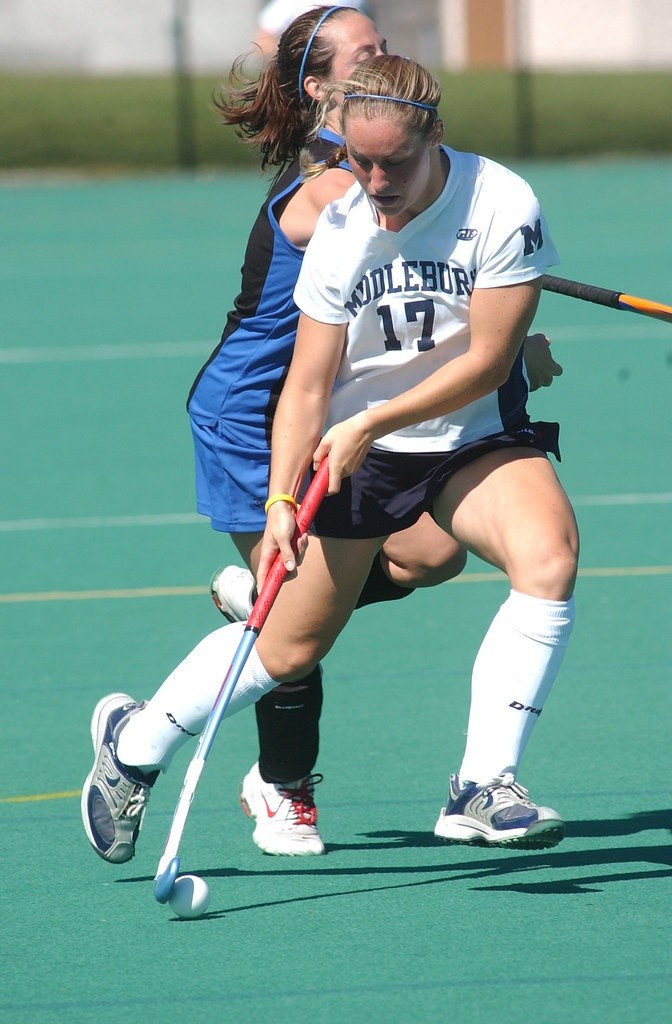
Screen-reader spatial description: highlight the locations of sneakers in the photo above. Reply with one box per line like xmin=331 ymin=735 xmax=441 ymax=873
xmin=241 ymin=762 xmax=325 ymax=856
xmin=81 ymin=693 xmax=160 ymax=864
xmin=210 ymin=565 xmax=258 ymax=623
xmin=434 ymin=772 xmax=566 ymax=850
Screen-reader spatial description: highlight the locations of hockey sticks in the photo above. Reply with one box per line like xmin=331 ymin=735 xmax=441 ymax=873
xmin=542 ymin=273 xmax=672 ymax=325
xmin=152 ymin=457 xmax=329 ymax=904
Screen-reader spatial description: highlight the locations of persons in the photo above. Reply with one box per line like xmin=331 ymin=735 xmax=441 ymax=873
xmin=184 ymin=5 xmax=565 ymax=857
xmin=78 ymin=52 xmax=580 ymax=863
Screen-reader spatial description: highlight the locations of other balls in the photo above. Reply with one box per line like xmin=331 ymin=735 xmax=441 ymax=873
xmin=168 ymin=875 xmax=211 ymax=919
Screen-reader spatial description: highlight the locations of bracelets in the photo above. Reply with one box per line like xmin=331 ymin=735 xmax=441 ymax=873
xmin=265 ymin=493 xmax=297 ymax=515
